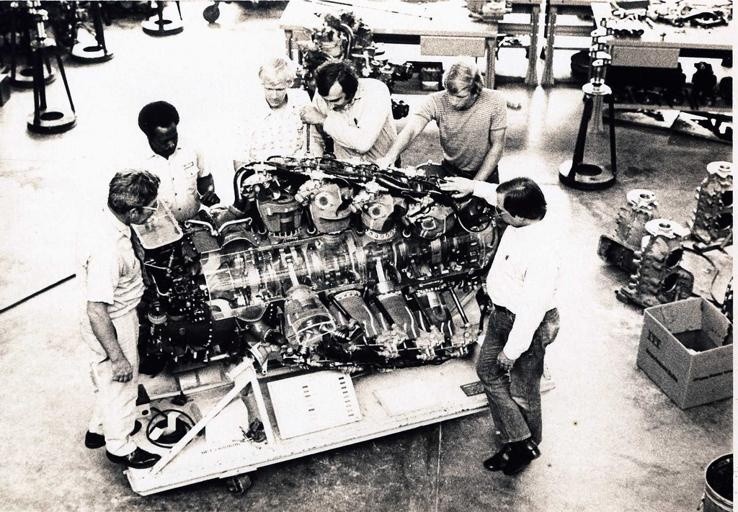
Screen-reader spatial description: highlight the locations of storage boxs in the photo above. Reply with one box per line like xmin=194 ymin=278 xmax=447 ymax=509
xmin=635 ymin=296 xmax=733 ymax=412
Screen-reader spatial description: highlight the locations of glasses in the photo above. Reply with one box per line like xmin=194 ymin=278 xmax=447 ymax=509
xmin=142 ymin=196 xmax=160 ymax=214
xmin=494 ymin=202 xmax=511 ymax=218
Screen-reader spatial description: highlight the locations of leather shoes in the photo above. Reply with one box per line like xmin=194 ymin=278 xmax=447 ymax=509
xmin=482 ymin=438 xmax=540 ymax=470
xmin=501 ymin=437 xmax=543 ymax=476
xmin=103 ymin=445 xmax=162 ymax=470
xmin=84 ymin=419 xmax=141 ymax=449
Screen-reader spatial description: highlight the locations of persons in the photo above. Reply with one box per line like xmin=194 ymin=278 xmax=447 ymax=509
xmin=374 ymin=54 xmax=509 ymax=186
xmin=436 ymin=175 xmax=560 ymax=476
xmin=299 ymin=63 xmax=403 ymax=169
xmin=130 ymin=101 xmax=221 ymax=228
xmin=230 ymin=53 xmax=326 ymax=176
xmin=78 ymin=167 xmax=163 ymax=469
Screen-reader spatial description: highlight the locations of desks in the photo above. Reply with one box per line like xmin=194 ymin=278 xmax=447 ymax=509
xmin=587 ymin=0 xmax=733 ymax=136
xmin=277 ymin=0 xmax=500 ymax=93
